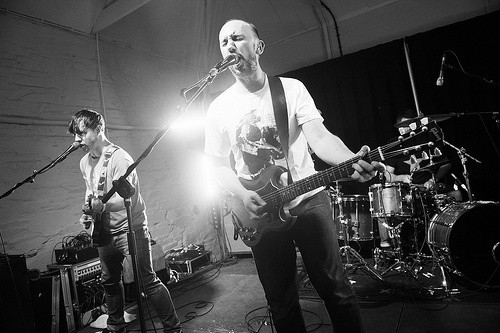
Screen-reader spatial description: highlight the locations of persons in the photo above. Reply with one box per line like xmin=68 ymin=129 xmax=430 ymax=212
xmin=386 ymin=108 xmax=462 ymax=201
xmin=203 ymin=18 xmax=387 ymax=333
xmin=66 ymin=107 xmax=182 ymax=333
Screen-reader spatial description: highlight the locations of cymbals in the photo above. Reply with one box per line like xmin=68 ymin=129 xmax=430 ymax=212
xmin=393 ymin=113 xmax=451 ymax=128
xmin=410 ymin=159 xmax=454 ymax=173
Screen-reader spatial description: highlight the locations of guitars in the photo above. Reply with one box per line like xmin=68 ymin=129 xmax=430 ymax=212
xmin=229 ymin=116 xmax=446 ymax=248
xmin=86 ymin=189 xmax=102 ymax=248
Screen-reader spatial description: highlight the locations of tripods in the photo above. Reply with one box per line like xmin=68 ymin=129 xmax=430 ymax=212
xmin=335 ymin=217 xmax=434 ymax=289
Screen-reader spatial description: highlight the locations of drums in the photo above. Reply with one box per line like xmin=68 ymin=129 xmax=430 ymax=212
xmin=328 ymin=194 xmax=380 ymax=241
xmin=427 ymin=201 xmax=500 ymax=287
xmin=367 ymin=182 xmax=413 ymax=217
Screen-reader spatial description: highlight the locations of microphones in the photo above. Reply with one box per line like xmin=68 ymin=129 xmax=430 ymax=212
xmin=437 ymin=54 xmax=447 ymax=86
xmin=50 ymin=142 xmax=80 ymax=167
xmin=209 ymin=54 xmax=241 ymax=76
